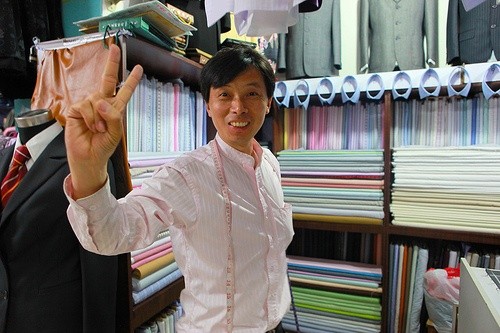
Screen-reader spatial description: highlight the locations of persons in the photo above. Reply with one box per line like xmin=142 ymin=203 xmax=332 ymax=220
xmin=63 ymin=44 xmax=295 ymax=333
xmin=0 ymin=108 xmax=131 ymax=332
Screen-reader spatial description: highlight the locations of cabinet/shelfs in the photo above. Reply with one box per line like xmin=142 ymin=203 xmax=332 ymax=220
xmin=31 ymin=28 xmax=500 ymax=333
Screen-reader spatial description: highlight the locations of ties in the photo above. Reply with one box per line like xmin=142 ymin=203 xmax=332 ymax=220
xmin=0 ymin=145 xmax=31 ymax=210
